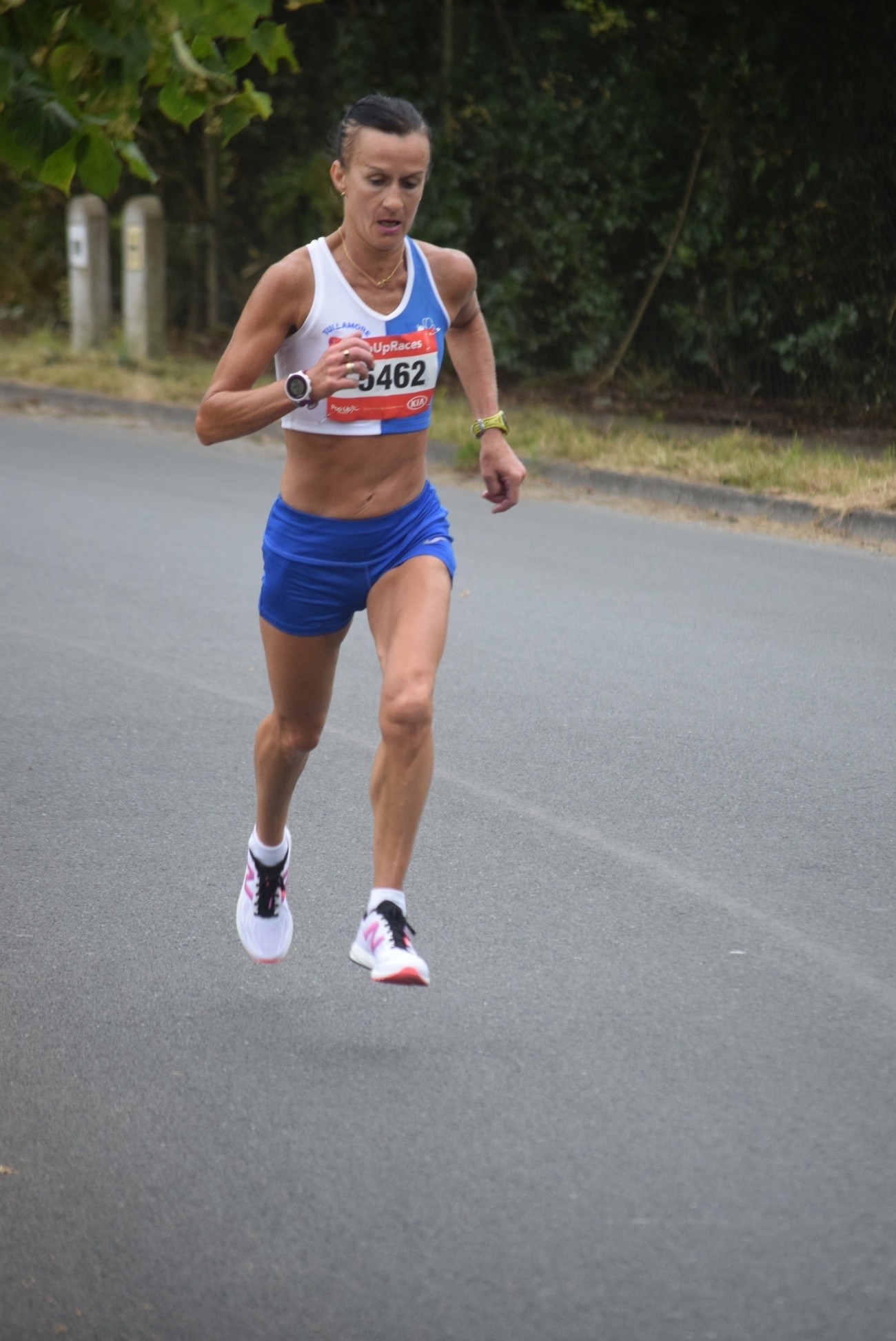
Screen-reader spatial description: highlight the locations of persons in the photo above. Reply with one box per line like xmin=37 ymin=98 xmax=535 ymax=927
xmin=194 ymin=98 xmax=527 ymax=986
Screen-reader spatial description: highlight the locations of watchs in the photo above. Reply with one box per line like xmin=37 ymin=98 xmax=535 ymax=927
xmin=471 ymin=410 xmax=510 ymax=439
xmin=282 ymin=371 xmax=317 ymax=410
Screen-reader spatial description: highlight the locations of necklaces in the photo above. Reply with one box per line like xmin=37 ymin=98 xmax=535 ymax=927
xmin=339 ymin=228 xmax=404 ymax=286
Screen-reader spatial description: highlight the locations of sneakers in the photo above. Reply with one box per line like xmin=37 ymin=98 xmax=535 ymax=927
xmin=236 ymin=823 xmax=293 ymax=962
xmin=348 ymin=900 xmax=431 ymax=987
